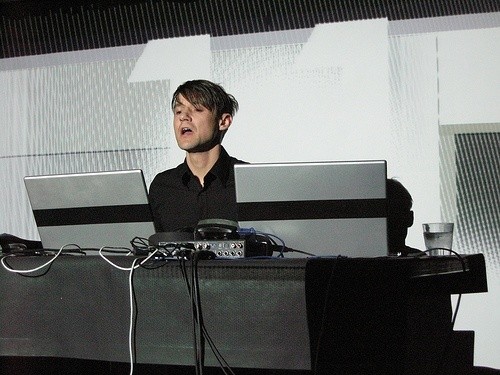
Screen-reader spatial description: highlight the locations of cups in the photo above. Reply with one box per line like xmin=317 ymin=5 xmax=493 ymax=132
xmin=422 ymin=221 xmax=454 ymax=258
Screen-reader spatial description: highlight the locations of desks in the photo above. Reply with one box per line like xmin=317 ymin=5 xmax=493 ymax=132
xmin=0 ymin=254 xmax=487 ymax=374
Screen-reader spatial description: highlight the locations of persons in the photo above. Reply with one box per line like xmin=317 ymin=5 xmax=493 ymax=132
xmin=147 ymin=78 xmax=253 ymax=234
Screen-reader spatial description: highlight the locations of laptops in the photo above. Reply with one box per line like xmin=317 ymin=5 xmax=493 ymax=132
xmin=233 ymin=159 xmax=389 ymax=258
xmin=23 ymin=169 xmax=156 ymax=257
